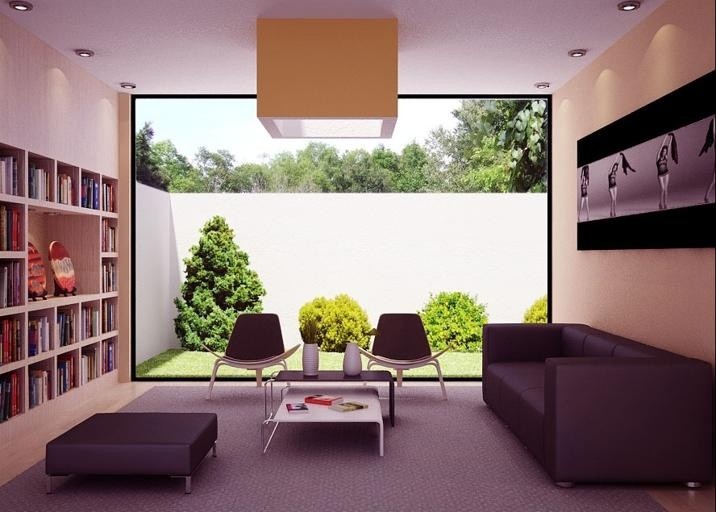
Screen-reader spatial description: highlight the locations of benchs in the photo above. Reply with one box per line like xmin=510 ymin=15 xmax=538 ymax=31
xmin=44 ymin=411 xmax=217 ymax=496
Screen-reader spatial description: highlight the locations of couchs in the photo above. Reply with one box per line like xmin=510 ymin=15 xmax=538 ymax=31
xmin=481 ymin=322 xmax=714 ymax=490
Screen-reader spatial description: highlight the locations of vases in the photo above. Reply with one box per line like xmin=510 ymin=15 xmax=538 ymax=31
xmin=343 ymin=342 xmax=363 ymax=378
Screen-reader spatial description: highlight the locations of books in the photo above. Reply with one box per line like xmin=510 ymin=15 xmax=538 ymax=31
xmin=21 ymin=299 xmax=102 ymax=409
xmin=0 ymin=150 xmax=116 ymax=213
xmin=0 ymin=203 xmax=24 ymax=422
xmin=305 ymin=393 xmax=343 ymax=405
xmin=98 ymin=216 xmax=116 ymax=374
xmin=285 ymin=402 xmax=309 ymax=413
xmin=330 ymin=400 xmax=369 ymax=413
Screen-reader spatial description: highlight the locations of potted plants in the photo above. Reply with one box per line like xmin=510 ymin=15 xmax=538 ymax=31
xmin=297 ymin=315 xmax=324 ymax=376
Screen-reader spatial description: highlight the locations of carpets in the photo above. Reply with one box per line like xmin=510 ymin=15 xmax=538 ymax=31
xmin=0 ymin=384 xmax=669 ymax=512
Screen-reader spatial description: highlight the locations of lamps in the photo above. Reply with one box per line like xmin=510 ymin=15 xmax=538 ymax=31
xmin=255 ymin=14 xmax=401 ymax=139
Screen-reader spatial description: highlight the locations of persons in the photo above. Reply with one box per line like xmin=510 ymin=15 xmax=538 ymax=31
xmin=607 ymin=154 xmax=622 ymax=217
xmin=704 ymin=170 xmax=715 ymax=203
xmin=656 ymin=134 xmax=672 ymax=211
xmin=578 ymin=166 xmax=592 ymax=222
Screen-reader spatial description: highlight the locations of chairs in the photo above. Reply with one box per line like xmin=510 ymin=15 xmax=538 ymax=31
xmin=359 ymin=310 xmax=456 ymax=404
xmin=203 ymin=310 xmax=301 ymax=404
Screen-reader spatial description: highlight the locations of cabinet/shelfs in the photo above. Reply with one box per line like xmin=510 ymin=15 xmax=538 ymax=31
xmin=0 ymin=141 xmax=118 ymax=424
